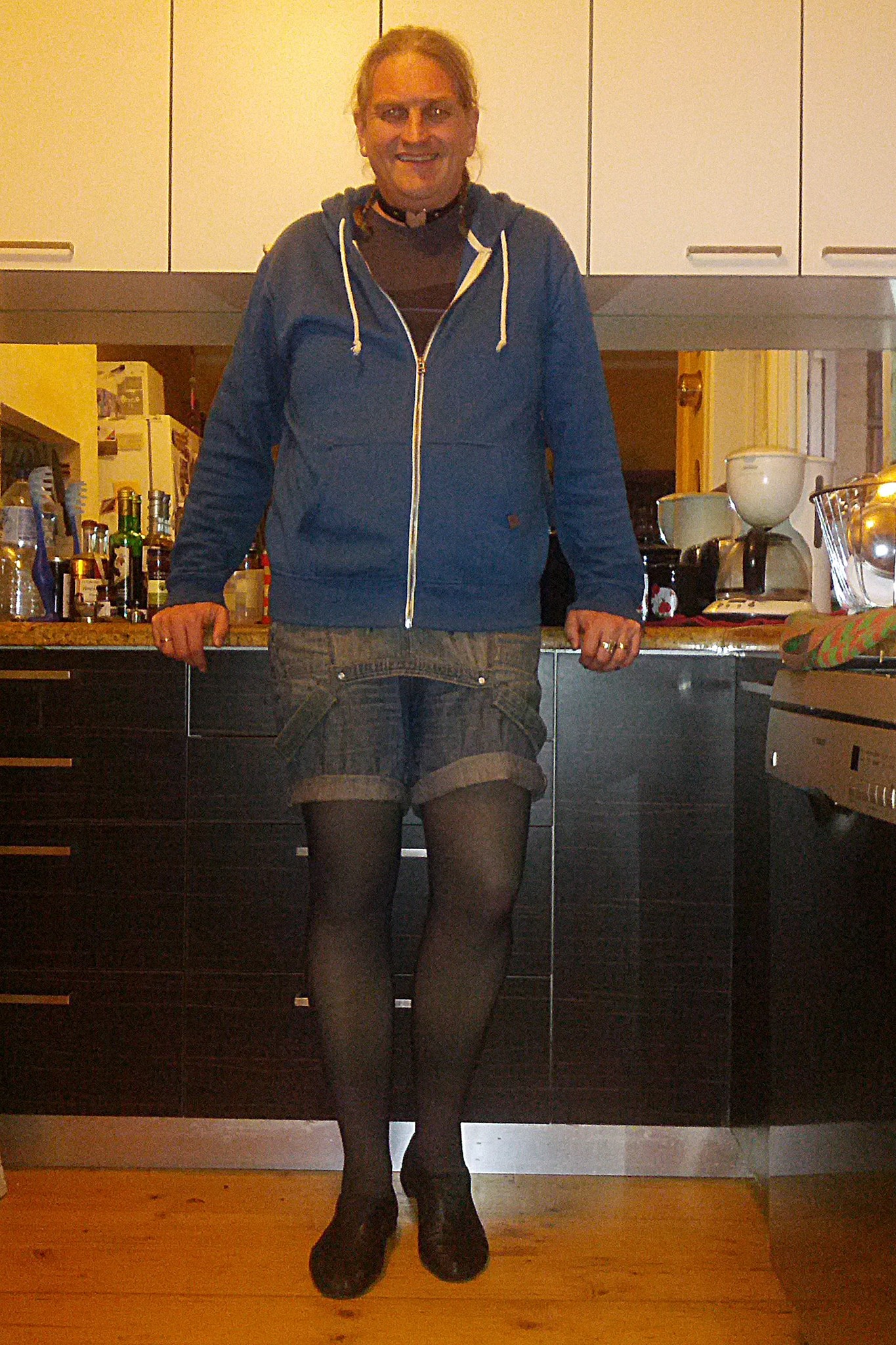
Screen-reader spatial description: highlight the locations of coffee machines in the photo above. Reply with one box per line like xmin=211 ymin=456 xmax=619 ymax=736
xmin=656 ymin=494 xmax=742 ymax=623
xmin=704 ymin=445 xmax=838 ymax=618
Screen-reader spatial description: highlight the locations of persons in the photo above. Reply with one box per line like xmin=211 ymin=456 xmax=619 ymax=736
xmin=150 ymin=27 xmax=645 ymax=1303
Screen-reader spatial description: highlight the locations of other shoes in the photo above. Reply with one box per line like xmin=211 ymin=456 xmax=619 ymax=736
xmin=400 ymin=1146 xmax=489 ymax=1281
xmin=308 ymin=1185 xmax=399 ymax=1298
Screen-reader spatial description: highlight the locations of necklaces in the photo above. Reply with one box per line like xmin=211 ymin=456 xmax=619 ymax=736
xmin=375 ymin=180 xmax=459 ymax=229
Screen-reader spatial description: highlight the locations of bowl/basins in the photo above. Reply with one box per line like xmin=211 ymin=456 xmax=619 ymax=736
xmin=845 ymin=554 xmax=895 ymax=607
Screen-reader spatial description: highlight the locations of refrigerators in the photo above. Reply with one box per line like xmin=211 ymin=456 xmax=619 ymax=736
xmin=98 ymin=415 xmax=203 ymax=538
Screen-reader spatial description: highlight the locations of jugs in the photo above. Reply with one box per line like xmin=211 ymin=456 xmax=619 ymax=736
xmin=683 ymin=537 xmax=732 ymax=608
xmin=715 ymin=531 xmax=810 ymax=601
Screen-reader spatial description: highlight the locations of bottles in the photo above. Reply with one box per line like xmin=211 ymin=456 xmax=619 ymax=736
xmin=1 ymin=486 xmax=185 ymax=621
xmin=224 ymin=545 xmax=265 ymax=626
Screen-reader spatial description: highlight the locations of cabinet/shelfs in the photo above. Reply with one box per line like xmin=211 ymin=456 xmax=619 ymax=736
xmin=0 ymin=0 xmax=896 ymax=365
xmin=0 ymin=618 xmax=746 ymax=1185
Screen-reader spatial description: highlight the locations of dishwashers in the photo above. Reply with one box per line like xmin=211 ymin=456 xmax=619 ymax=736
xmin=761 ymin=665 xmax=896 ymax=1343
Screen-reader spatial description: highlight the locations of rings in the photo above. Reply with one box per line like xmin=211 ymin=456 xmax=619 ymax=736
xmin=598 ymin=642 xmax=618 ymax=651
xmin=160 ymin=636 xmax=173 ymax=643
xmin=615 ymin=642 xmax=631 ymax=650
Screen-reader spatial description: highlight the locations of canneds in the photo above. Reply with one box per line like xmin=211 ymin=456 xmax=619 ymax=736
xmin=223 ymin=547 xmax=266 ymax=627
xmin=48 ymin=556 xmax=72 ymax=621
xmin=147 ymin=547 xmax=171 ymax=622
